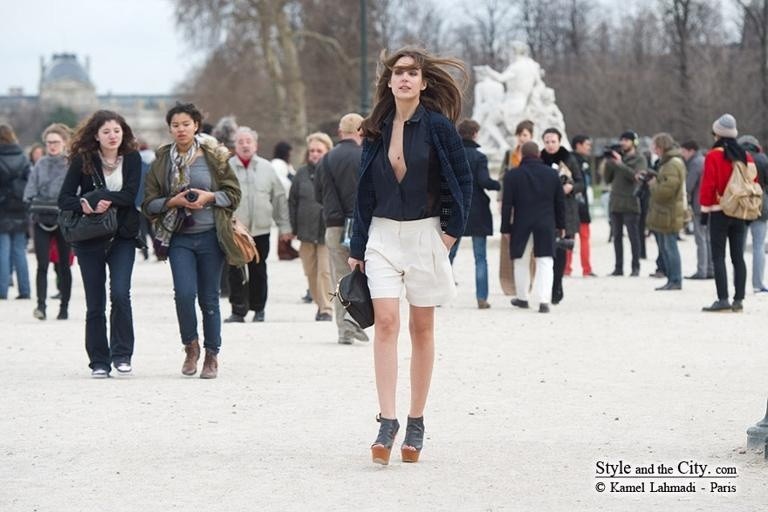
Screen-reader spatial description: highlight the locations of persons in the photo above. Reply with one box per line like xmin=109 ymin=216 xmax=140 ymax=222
xmin=0 ymin=110 xmax=768 ymax=323
xmin=144 ymin=100 xmax=243 ymax=381
xmin=472 ymin=41 xmax=566 ymax=152
xmin=347 ymin=44 xmax=474 ymax=468
xmin=55 ymin=107 xmax=144 ymax=382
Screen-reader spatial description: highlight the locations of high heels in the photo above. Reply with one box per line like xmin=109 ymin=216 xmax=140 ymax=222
xmin=402 ymin=414 xmax=424 ymax=462
xmin=371 ymin=413 xmax=399 ymax=464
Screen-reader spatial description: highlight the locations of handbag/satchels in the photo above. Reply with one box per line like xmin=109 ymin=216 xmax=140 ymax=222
xmin=337 ymin=264 xmax=374 ymax=328
xmin=31 ymin=196 xmax=62 ymax=230
xmin=229 ymin=219 xmax=258 ymax=265
xmin=57 ymin=208 xmax=120 ymax=242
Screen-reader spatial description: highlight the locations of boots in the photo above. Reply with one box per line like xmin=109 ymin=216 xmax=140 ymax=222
xmin=201 ymin=347 xmax=218 ymax=379
xmin=182 ymin=341 xmax=200 ymax=375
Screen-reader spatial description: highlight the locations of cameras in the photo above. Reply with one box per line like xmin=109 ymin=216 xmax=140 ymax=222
xmin=186 ymin=190 xmax=198 ymax=202
xmin=633 ymin=174 xmax=648 ymax=198
xmin=554 ymin=236 xmax=575 ymax=251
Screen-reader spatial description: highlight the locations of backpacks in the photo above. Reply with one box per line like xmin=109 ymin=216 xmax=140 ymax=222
xmin=1 ymin=154 xmax=28 ymax=211
xmin=713 ymin=147 xmax=763 ymax=221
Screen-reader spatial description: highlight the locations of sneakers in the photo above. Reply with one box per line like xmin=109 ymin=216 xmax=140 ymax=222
xmin=339 ymin=319 xmax=370 ymax=345
xmin=92 ymin=361 xmax=130 ymax=378
xmin=511 ymin=297 xmax=528 ymax=307
xmin=648 ymin=272 xmax=680 ymax=290
xmin=539 ymin=303 xmax=549 ymax=311
xmin=703 ymin=300 xmax=743 ymax=313
xmin=34 ymin=304 xmax=68 ymax=320
xmin=684 ymin=274 xmax=714 ymax=279
xmin=317 ymin=308 xmax=331 ymax=321
xmin=224 ymin=310 xmax=263 ymax=322
xmin=478 ymin=301 xmax=490 ymax=309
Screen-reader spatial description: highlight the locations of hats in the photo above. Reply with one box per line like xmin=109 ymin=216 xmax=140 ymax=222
xmin=739 ymin=134 xmax=762 ymax=152
xmin=712 ymin=114 xmax=738 ymax=138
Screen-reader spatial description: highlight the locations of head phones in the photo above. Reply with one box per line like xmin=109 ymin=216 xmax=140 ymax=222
xmin=627 ymin=131 xmax=640 ymax=147
